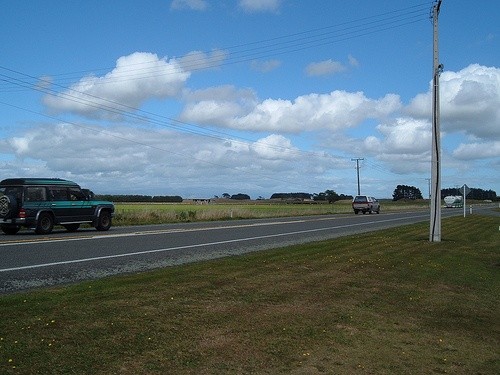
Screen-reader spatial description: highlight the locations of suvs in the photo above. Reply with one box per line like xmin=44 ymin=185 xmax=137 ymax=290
xmin=0 ymin=178 xmax=115 ymax=234
xmin=352 ymin=195 xmax=380 ymax=215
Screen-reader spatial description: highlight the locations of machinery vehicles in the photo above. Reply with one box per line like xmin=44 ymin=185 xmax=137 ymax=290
xmin=445 ymin=196 xmax=464 ymax=208
